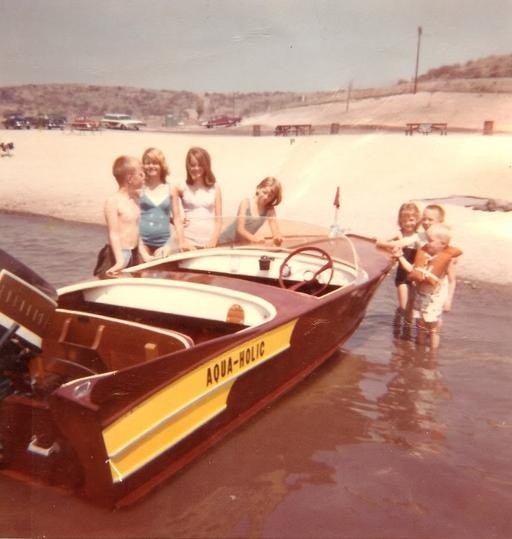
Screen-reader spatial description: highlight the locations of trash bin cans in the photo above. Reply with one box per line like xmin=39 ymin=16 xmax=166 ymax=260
xmin=483 ymin=120 xmax=493 ymax=135
xmin=331 ymin=123 xmax=340 ymax=134
xmin=253 ymin=125 xmax=260 ymax=135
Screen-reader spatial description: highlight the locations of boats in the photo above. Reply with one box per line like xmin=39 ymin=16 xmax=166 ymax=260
xmin=0 ymin=235 xmax=396 ymax=514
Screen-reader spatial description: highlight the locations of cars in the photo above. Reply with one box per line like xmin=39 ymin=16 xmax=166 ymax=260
xmin=202 ymin=115 xmax=243 ymax=128
xmin=3 ymin=113 xmax=148 ymax=131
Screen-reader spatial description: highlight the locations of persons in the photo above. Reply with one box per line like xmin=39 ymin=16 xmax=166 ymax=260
xmin=376 ymin=203 xmax=457 ymax=348
xmin=93 ymin=154 xmax=156 ymax=276
xmin=153 ymin=146 xmax=223 ymax=258
xmin=134 ymin=147 xmax=192 ymax=253
xmin=217 ymin=175 xmax=284 ymax=247
xmin=370 ymin=349 xmax=443 ymax=456
